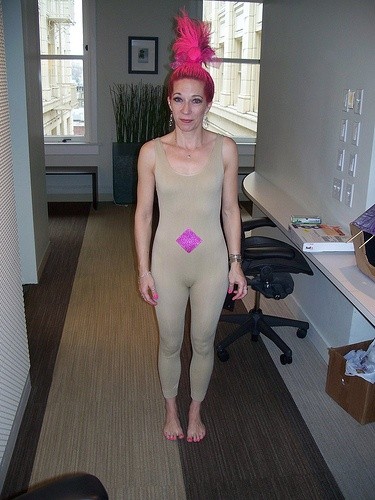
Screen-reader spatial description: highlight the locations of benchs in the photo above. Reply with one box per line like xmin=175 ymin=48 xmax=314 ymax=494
xmin=45 ymin=166 xmax=98 ymax=210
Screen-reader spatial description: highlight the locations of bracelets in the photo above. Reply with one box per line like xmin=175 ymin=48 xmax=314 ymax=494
xmin=138 ymin=271 xmax=151 ymax=279
xmin=245 ymin=286 xmax=248 ymax=290
xmin=228 ymin=254 xmax=242 ymax=265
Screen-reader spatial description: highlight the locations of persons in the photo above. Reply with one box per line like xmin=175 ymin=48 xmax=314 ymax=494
xmin=137 ymin=9 xmax=249 ymax=441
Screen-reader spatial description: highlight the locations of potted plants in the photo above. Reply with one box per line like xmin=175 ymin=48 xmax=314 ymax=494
xmin=109 ymin=82 xmax=174 ymax=206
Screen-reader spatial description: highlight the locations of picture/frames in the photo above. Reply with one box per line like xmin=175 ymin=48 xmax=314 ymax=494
xmin=128 ymin=36 xmax=159 ymax=74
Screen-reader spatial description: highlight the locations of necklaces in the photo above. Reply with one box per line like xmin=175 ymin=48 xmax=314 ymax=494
xmin=187 ymin=152 xmax=192 ymax=159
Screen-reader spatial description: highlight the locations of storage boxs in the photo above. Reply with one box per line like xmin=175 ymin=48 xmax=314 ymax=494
xmin=324 ymin=340 xmax=375 ymax=424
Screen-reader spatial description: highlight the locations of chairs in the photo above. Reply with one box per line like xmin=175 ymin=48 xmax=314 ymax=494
xmin=214 ymin=212 xmax=312 ymax=365
